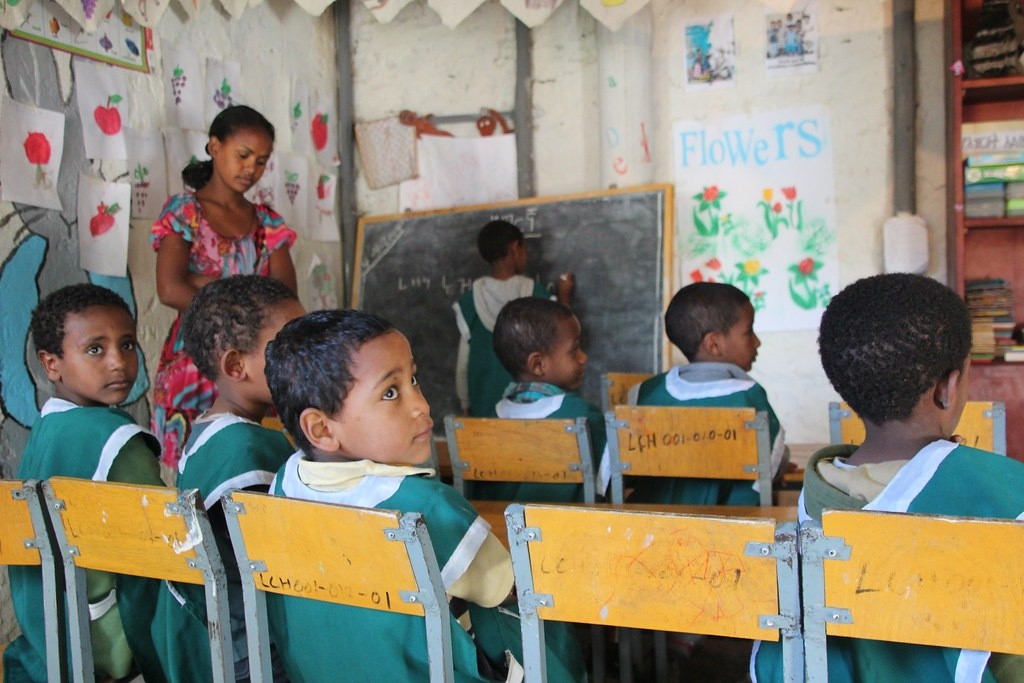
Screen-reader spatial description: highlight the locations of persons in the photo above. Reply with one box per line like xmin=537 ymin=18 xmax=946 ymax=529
xmin=478 ymin=296 xmax=608 ymax=505
xmin=766 ymin=13 xmax=814 ymax=57
xmin=622 ymin=281 xmax=790 ymax=661
xmin=150 ymin=273 xmax=308 ymax=683
xmin=7 ymin=283 xmax=171 ymax=683
xmin=689 ymin=48 xmax=732 ymax=81
xmin=263 ymin=307 xmax=587 ymax=683
xmin=752 ymin=272 xmax=1024 ymax=683
xmin=452 ymin=219 xmax=574 ymax=417
xmin=148 ymin=105 xmax=297 ymax=472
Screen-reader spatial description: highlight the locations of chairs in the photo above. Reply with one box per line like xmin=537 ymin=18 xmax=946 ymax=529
xmin=444 ymin=374 xmax=1024 ymax=683
xmin=1 ymin=479 xmax=69 ymax=683
xmin=222 ymin=488 xmax=455 ymax=683
xmin=40 ymin=476 xmax=237 ymax=683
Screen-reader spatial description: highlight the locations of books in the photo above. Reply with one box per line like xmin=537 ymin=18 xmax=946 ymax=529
xmin=965 ymin=276 xmax=1024 ymax=364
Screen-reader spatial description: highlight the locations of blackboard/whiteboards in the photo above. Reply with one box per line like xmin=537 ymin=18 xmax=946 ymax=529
xmin=351 ymin=183 xmax=675 ymax=469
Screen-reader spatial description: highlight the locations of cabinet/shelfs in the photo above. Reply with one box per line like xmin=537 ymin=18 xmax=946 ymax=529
xmin=942 ymin=0 xmax=1023 ymax=461
